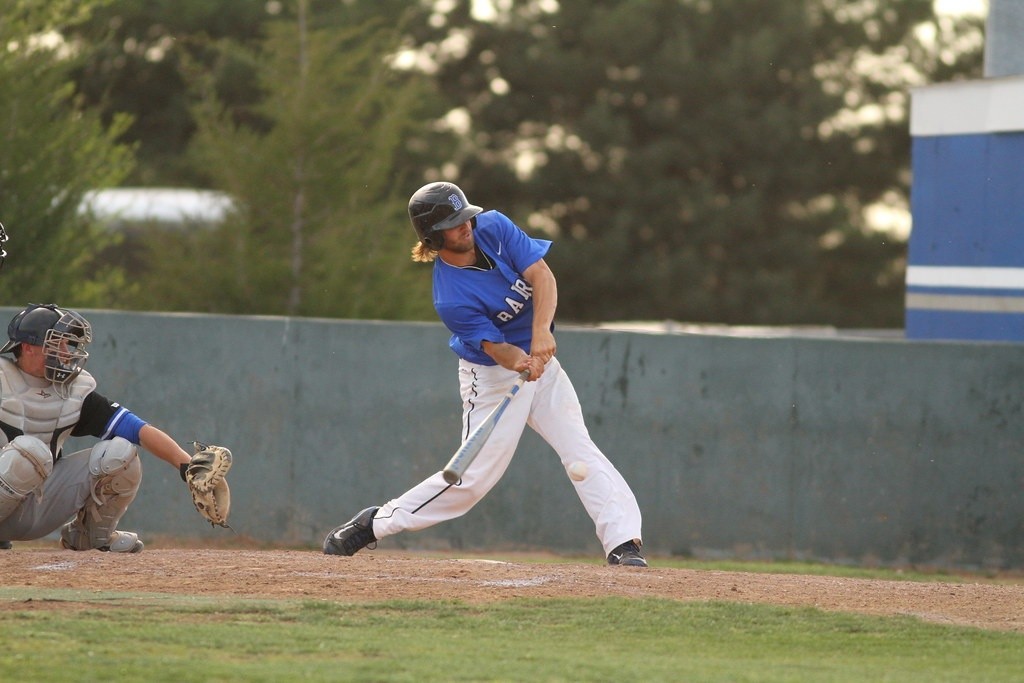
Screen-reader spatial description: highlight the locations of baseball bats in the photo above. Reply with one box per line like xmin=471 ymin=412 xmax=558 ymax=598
xmin=442 ymin=367 xmax=532 ymax=484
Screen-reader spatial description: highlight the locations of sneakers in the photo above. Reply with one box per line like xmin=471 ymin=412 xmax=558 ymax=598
xmin=608 ymin=540 xmax=648 ymax=567
xmin=0 ymin=541 xmax=12 ymax=549
xmin=323 ymin=506 xmax=381 ymax=556
xmin=60 ymin=531 xmax=144 ymax=553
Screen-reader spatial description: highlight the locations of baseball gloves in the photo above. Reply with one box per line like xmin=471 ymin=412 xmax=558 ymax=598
xmin=185 ymin=445 xmax=234 ymax=527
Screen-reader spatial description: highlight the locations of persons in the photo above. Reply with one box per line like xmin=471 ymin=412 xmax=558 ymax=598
xmin=323 ymin=181 xmax=647 ymax=566
xmin=0 ymin=304 xmax=233 ymax=554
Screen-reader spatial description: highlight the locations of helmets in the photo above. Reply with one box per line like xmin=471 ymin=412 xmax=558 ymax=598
xmin=408 ymin=181 xmax=484 ymax=250
xmin=0 ymin=307 xmax=84 ymax=354
